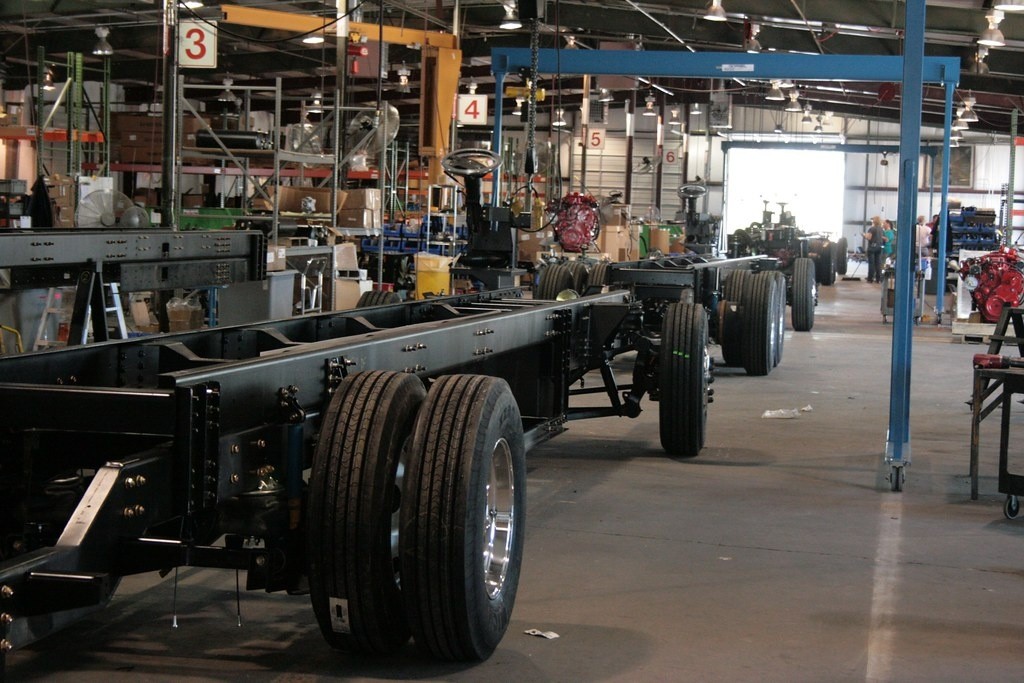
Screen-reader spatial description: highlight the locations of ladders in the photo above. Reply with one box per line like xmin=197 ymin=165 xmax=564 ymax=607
xmin=34 ymin=283 xmax=128 ymax=353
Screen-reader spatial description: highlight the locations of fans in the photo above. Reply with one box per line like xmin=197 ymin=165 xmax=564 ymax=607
xmin=633 ymin=156 xmax=662 ymax=175
xmin=74 ymin=187 xmax=133 ymax=229
xmin=347 ymin=101 xmax=400 ymax=156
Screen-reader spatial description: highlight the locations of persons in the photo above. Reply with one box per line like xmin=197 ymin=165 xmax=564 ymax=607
xmin=861 ymin=215 xmax=895 ymax=283
xmin=912 ymin=216 xmax=938 ymax=255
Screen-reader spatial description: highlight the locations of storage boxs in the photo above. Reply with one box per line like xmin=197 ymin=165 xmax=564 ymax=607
xmin=298 ymin=277 xmax=373 ymax=311
xmin=137 ymin=309 xmax=202 ymax=334
xmin=338 ymin=189 xmax=381 ymax=229
xmin=519 ymin=205 xmax=641 ymax=261
xmin=139 ymin=187 xmax=205 ymax=208
xmin=268 ymin=244 xmax=286 ymax=270
xmin=1 ymin=174 xmax=76 ymax=228
xmin=107 ymin=111 xmax=238 ymax=165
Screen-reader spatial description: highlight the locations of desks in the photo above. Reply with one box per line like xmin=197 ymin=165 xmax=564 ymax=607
xmin=971 ymin=366 xmax=1024 ymax=519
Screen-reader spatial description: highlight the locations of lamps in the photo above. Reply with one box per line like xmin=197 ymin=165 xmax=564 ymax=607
xmin=396 ymin=60 xmax=411 ymax=92
xmin=564 ymin=36 xmax=580 ymax=50
xmin=511 ymin=97 xmax=524 ymax=116
xmin=92 ymin=25 xmax=113 ymax=55
xmin=775 ymin=125 xmax=783 ymax=134
xmin=690 ymin=103 xmax=702 ymax=115
xmin=970 ymin=46 xmax=990 ymax=74
xmin=499 ymin=0 xmax=522 ymax=30
xmin=765 ymin=79 xmax=794 ymax=101
xmin=950 ymin=131 xmax=963 ymax=148
xmin=308 ymin=89 xmax=324 ymax=113
xmin=747 ymin=24 xmax=762 ymax=53
xmin=959 ymin=89 xmax=978 ymax=122
xmin=668 ymin=105 xmax=681 ymax=125
xmin=302 ymin=33 xmax=325 ymax=44
xmin=991 ymin=0 xmax=1024 ymax=12
xmin=703 ymin=0 xmax=727 ymax=21
xmin=802 ymin=100 xmax=812 ymax=123
xmin=814 ymin=111 xmax=823 ymax=133
xmin=952 ymin=107 xmax=969 ymax=130
xmin=218 ymin=71 xmax=238 ymax=101
xmin=42 ymin=65 xmax=55 ymax=90
xmin=552 ymin=109 xmax=566 ymax=126
xmin=598 ymin=90 xmax=614 ymax=102
xmin=642 ymin=91 xmax=656 ymax=116
xmin=785 ymin=90 xmax=802 ymax=111
xmin=977 ymin=9 xmax=1006 ymax=46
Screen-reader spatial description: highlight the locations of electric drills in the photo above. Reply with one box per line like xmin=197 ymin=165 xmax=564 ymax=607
xmin=972 ymin=354 xmax=1024 ymax=371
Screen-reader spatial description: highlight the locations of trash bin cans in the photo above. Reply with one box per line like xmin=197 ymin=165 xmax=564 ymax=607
xmin=414 ymin=252 xmax=454 ymax=299
